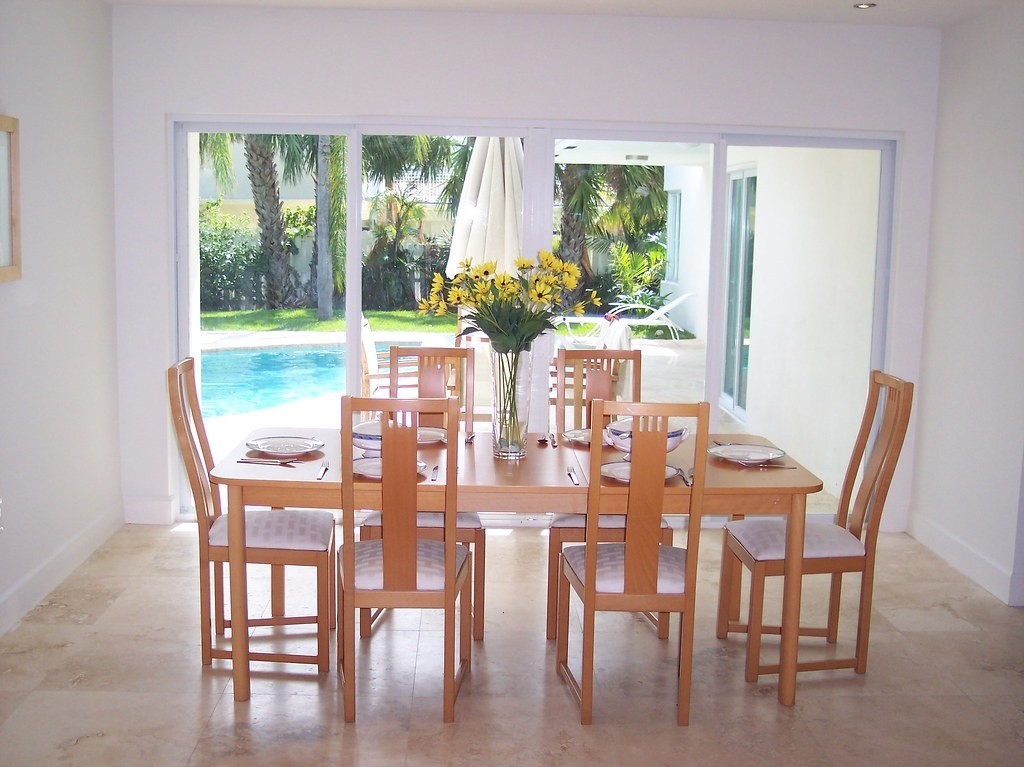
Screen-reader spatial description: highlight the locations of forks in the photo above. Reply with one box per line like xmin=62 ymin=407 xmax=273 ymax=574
xmin=466 ymin=434 xmax=474 ymax=443
xmin=316 ymin=460 xmax=329 ymax=479
xmin=237 ymin=459 xmax=303 ymax=465
xmin=566 ymin=465 xmax=579 ymax=484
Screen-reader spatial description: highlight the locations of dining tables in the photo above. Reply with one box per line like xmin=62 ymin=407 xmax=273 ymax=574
xmin=210 ymin=426 xmax=823 ymax=708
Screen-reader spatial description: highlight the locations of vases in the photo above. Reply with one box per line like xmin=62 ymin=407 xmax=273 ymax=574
xmin=489 ymin=340 xmax=534 ymax=461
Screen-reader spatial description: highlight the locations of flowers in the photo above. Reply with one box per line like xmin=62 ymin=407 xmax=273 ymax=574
xmin=416 ymin=248 xmax=603 ymax=451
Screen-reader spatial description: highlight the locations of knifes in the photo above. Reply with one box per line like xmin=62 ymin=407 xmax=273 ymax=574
xmin=431 ymin=466 xmax=438 ymax=480
xmin=549 ymin=433 xmax=558 ymax=447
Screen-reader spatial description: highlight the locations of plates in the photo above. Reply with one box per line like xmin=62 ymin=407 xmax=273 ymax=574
xmin=353 ymin=458 xmax=427 ymax=479
xmin=563 ymin=428 xmax=608 ymax=446
xmin=707 ymin=444 xmax=785 ymax=463
xmin=246 ymin=436 xmax=324 ymax=455
xmin=601 ymin=462 xmax=680 ymax=482
xmin=417 ymin=427 xmax=447 ymax=444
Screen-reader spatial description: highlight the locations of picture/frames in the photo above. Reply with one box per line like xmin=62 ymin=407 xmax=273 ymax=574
xmin=0 ymin=114 xmax=23 ymax=282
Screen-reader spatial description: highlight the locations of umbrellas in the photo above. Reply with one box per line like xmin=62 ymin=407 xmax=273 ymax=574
xmin=446 ymin=135 xmax=526 ymax=288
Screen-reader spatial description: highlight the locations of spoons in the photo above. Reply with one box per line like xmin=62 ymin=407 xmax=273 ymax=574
xmin=738 ymin=460 xmax=797 ymax=469
xmin=679 ymin=468 xmax=690 ymax=486
xmin=538 ymin=434 xmax=548 ymax=443
xmin=713 ymin=441 xmax=768 ymax=446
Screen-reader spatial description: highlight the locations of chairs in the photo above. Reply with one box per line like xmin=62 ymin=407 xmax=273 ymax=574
xmin=548 ymin=316 xmax=628 ymax=433
xmin=555 ymin=398 xmax=711 ymax=728
xmin=546 ymin=349 xmax=673 ymax=642
xmin=360 ymin=318 xmax=463 ymax=432
xmin=167 ymin=356 xmax=337 ymax=674
xmin=335 ymin=392 xmax=473 ymax=724
xmin=358 ymin=345 xmax=486 ymax=641
xmin=715 ymin=369 xmax=915 ymax=706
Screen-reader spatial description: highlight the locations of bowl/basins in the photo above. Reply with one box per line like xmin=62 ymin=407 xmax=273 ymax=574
xmin=606 ymin=416 xmax=689 ymax=461
xmin=352 ymin=420 xmax=402 ymax=458
